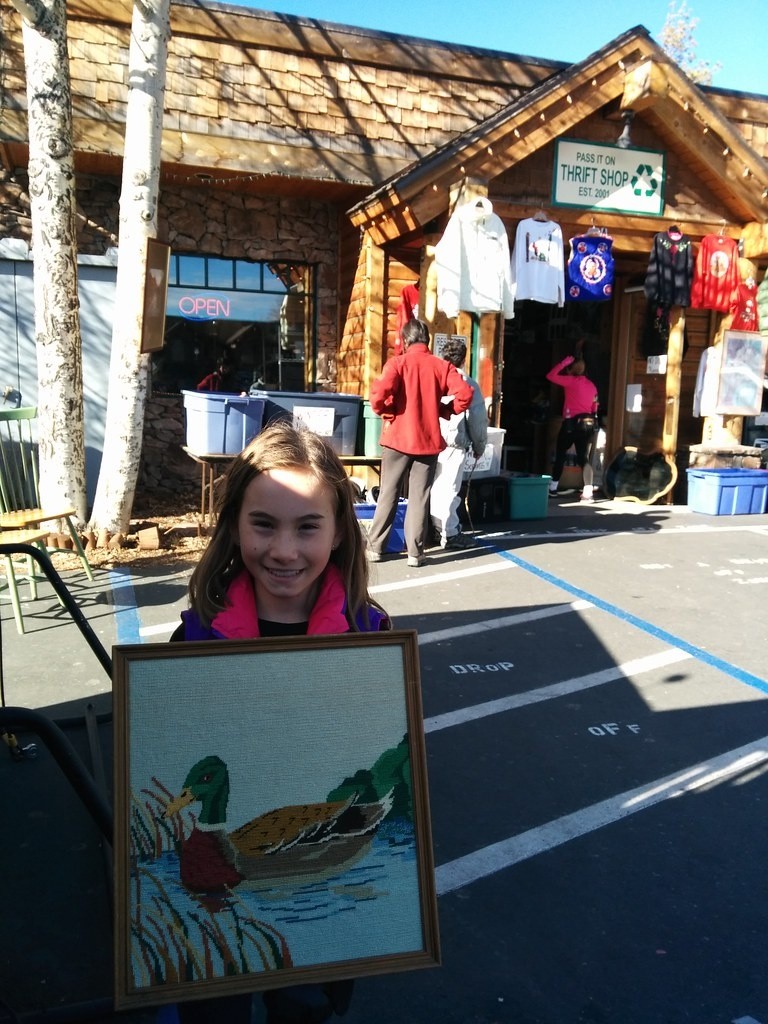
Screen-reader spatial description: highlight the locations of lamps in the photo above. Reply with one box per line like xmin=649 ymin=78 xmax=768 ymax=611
xmin=618 ymin=109 xmax=635 ymax=151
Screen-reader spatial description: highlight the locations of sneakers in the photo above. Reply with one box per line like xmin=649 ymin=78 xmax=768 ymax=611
xmin=439 ymin=525 xmax=478 ymax=549
xmin=427 ymin=525 xmax=441 ymax=544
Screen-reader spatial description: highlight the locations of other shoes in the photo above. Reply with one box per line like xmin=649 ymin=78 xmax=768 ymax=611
xmin=548 ymin=488 xmax=558 ymax=498
xmin=570 ymin=490 xmax=594 ymax=503
xmin=365 ymin=550 xmax=382 ymax=563
xmin=406 ymin=554 xmax=426 ymax=568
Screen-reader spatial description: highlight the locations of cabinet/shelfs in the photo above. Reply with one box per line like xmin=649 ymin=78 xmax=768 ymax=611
xmin=530 ymin=382 xmax=561 ymax=474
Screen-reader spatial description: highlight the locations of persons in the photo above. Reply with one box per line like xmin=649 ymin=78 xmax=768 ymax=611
xmin=197 ymin=358 xmax=239 ymax=391
xmin=430 ymin=340 xmax=487 ymax=548
xmin=546 ymin=355 xmax=600 ymax=503
xmin=364 ymin=319 xmax=474 ymax=566
xmin=167 ymin=425 xmax=391 ymax=1024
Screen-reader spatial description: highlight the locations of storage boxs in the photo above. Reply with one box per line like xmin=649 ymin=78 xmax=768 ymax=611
xmin=686 ymin=469 xmax=768 ymax=517
xmin=546 ymin=465 xmax=583 ymax=490
xmin=250 ymin=390 xmax=364 ymax=457
xmin=479 ymin=471 xmax=551 ymax=523
xmin=180 ymin=389 xmax=266 ymax=456
xmin=354 ymin=400 xmax=383 ymax=458
xmin=352 ymin=496 xmax=408 ymax=555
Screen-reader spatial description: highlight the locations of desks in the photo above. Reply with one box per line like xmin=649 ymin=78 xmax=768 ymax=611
xmin=179 ymin=444 xmax=383 ymax=540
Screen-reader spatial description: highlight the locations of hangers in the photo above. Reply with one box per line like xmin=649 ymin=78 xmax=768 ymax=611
xmin=532 ymin=201 xmax=548 ymax=222
xmin=718 ymin=218 xmax=726 ymax=238
xmin=475 ymin=201 xmax=482 ymax=207
xmin=587 ymin=218 xmax=600 ymax=234
xmin=744 ymin=269 xmax=754 ymax=289
xmin=670 ymin=219 xmax=681 ymax=235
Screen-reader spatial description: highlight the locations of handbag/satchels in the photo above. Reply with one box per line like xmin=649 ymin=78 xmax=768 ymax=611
xmin=574 ymin=411 xmax=598 ymax=434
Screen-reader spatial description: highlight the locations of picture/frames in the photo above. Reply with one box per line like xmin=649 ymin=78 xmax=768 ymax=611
xmin=109 ymin=629 xmax=442 ymax=1016
xmin=714 ymin=329 xmax=768 ymax=418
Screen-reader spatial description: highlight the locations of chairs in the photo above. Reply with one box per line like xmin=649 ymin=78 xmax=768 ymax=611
xmin=0 ymin=529 xmax=67 ymax=636
xmin=0 ymin=406 xmax=95 ymax=600
